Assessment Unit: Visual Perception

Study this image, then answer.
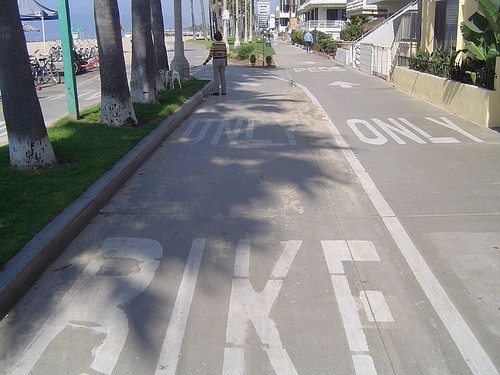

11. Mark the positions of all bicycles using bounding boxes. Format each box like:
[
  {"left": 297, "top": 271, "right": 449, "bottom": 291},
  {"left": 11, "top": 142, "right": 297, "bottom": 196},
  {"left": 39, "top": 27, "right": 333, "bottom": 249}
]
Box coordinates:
[{"left": 28, "top": 43, "right": 99, "bottom": 91}]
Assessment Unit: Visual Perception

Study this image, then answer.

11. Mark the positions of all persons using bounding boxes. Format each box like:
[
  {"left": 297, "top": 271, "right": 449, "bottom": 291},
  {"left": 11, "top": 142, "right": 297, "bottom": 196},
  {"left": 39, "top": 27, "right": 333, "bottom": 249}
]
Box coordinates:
[
  {"left": 202, "top": 31, "right": 228, "bottom": 96},
  {"left": 273, "top": 27, "right": 278, "bottom": 46},
  {"left": 304, "top": 29, "right": 314, "bottom": 53}
]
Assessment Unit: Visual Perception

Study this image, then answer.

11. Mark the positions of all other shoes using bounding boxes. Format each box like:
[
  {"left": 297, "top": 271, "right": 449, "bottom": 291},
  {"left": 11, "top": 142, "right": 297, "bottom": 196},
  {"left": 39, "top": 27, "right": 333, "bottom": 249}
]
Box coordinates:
[
  {"left": 221, "top": 92, "right": 227, "bottom": 95},
  {"left": 210, "top": 92, "right": 220, "bottom": 97}
]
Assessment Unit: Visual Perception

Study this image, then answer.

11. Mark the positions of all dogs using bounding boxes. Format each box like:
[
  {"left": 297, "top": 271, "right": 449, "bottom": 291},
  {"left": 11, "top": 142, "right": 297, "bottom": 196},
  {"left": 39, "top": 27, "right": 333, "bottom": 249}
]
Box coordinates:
[{"left": 164, "top": 69, "right": 181, "bottom": 90}]
